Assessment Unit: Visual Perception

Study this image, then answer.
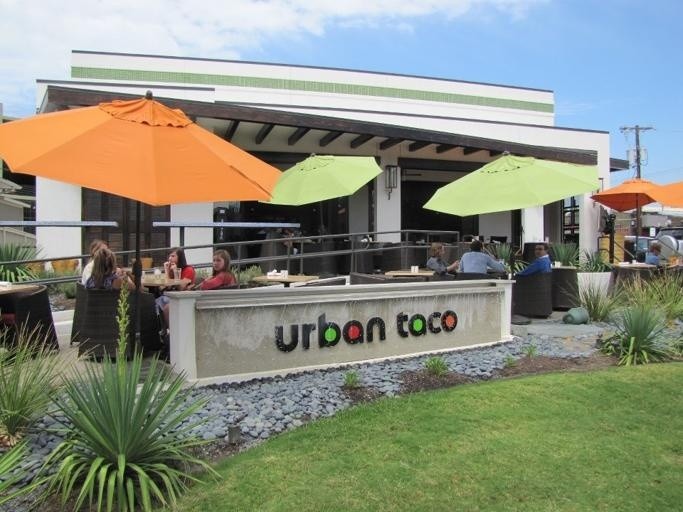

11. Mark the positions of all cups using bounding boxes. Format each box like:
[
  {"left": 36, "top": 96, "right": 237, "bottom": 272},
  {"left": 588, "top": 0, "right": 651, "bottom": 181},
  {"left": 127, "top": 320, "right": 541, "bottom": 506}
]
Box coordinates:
[
  {"left": 280, "top": 269, "right": 288, "bottom": 280},
  {"left": 114, "top": 268, "right": 126, "bottom": 277},
  {"left": 415, "top": 265, "right": 419, "bottom": 273},
  {"left": 142, "top": 270, "right": 161, "bottom": 282},
  {"left": 554, "top": 261, "right": 560, "bottom": 268},
  {"left": 173, "top": 267, "right": 181, "bottom": 281},
  {"left": 410, "top": 266, "right": 414, "bottom": 273},
  {"left": 632, "top": 260, "right": 636, "bottom": 264}
]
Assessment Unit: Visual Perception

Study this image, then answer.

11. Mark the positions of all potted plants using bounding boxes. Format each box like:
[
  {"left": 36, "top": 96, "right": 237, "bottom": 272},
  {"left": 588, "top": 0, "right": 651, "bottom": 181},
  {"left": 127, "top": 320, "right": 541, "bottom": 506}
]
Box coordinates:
[{"left": 578, "top": 249, "right": 611, "bottom": 310}]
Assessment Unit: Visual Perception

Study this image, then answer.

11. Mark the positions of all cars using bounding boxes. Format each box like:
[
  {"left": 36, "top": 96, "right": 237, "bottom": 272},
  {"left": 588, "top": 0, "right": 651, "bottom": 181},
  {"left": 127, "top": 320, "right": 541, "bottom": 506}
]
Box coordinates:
[{"left": 622, "top": 236, "right": 654, "bottom": 263}]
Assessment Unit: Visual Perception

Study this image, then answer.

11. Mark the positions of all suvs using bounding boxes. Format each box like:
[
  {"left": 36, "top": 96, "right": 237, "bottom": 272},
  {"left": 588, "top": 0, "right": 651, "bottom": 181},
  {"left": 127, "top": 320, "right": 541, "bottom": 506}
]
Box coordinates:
[{"left": 653, "top": 226, "right": 682, "bottom": 255}]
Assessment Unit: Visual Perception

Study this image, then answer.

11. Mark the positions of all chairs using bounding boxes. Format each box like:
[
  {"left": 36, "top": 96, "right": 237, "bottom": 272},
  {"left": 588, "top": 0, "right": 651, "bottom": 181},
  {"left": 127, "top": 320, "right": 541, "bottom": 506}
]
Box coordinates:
[
  {"left": 613, "top": 263, "right": 681, "bottom": 298},
  {"left": 352, "top": 271, "right": 425, "bottom": 290},
  {"left": 551, "top": 267, "right": 581, "bottom": 311},
  {"left": 0, "top": 272, "right": 249, "bottom": 361},
  {"left": 510, "top": 270, "right": 552, "bottom": 318},
  {"left": 289, "top": 277, "right": 348, "bottom": 291}
]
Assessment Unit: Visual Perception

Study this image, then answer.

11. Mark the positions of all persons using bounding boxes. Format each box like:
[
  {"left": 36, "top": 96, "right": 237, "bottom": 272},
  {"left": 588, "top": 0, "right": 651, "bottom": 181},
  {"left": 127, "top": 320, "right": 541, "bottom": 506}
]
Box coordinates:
[
  {"left": 515, "top": 243, "right": 552, "bottom": 274},
  {"left": 645, "top": 243, "right": 662, "bottom": 265},
  {"left": 426, "top": 241, "right": 460, "bottom": 274},
  {"left": 461, "top": 240, "right": 506, "bottom": 274},
  {"left": 80, "top": 240, "right": 237, "bottom": 327}
]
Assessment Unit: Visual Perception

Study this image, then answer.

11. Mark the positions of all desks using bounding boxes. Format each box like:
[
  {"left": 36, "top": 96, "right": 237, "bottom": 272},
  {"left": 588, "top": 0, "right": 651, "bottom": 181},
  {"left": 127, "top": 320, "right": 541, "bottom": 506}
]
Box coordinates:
[
  {"left": 256, "top": 272, "right": 317, "bottom": 284},
  {"left": 382, "top": 269, "right": 442, "bottom": 284}
]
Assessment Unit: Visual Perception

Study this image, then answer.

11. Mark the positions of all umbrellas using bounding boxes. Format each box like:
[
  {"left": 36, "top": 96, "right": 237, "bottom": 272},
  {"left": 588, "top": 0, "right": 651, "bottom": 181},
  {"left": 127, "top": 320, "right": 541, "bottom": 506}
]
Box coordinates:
[
  {"left": 258, "top": 153, "right": 384, "bottom": 235},
  {"left": 0, "top": 90, "right": 283, "bottom": 353},
  {"left": 422, "top": 152, "right": 600, "bottom": 218},
  {"left": 657, "top": 182, "right": 683, "bottom": 208},
  {"left": 591, "top": 179, "right": 660, "bottom": 249}
]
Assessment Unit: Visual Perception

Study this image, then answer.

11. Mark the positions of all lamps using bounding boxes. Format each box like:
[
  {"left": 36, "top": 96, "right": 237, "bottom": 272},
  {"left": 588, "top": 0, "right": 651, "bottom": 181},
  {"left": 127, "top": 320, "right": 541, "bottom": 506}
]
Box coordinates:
[{"left": 383, "top": 163, "right": 398, "bottom": 200}]
